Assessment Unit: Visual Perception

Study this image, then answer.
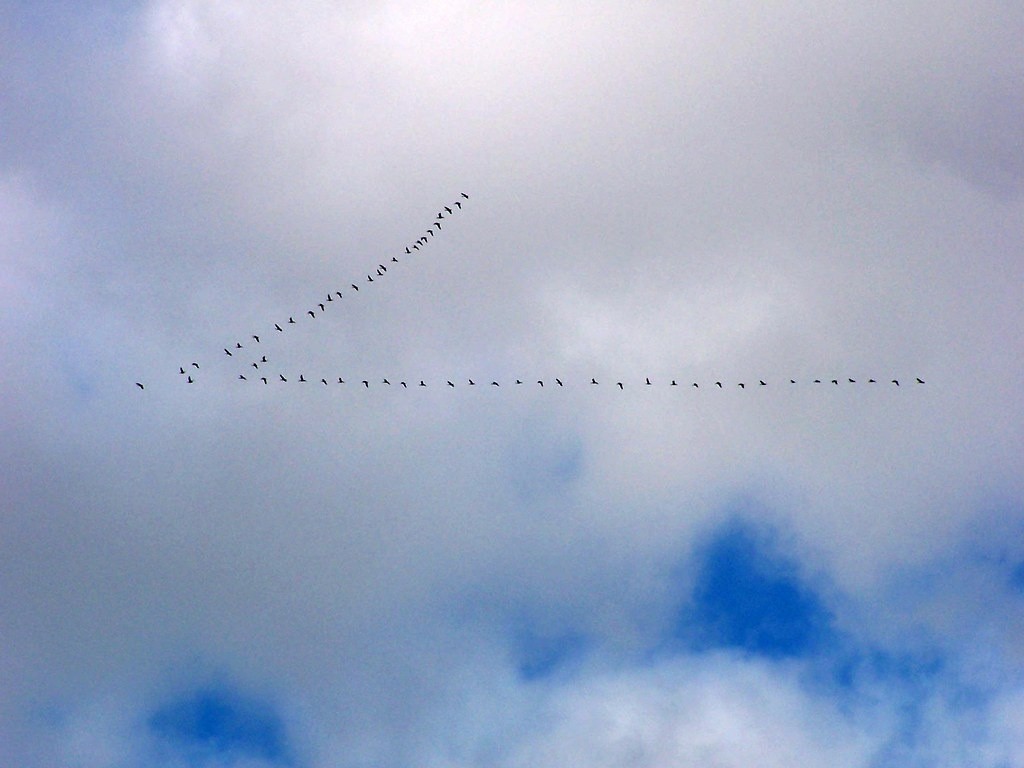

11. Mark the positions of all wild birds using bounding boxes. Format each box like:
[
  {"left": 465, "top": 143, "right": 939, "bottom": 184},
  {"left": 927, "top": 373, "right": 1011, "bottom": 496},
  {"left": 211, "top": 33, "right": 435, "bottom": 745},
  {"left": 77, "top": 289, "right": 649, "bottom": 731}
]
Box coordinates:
[
  {"left": 136, "top": 349, "right": 268, "bottom": 390},
  {"left": 278, "top": 374, "right": 926, "bottom": 390},
  {"left": 236, "top": 192, "right": 469, "bottom": 348}
]
